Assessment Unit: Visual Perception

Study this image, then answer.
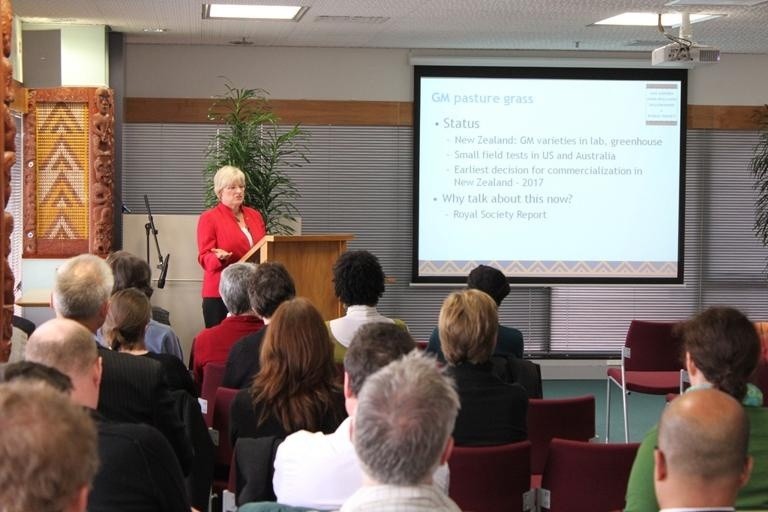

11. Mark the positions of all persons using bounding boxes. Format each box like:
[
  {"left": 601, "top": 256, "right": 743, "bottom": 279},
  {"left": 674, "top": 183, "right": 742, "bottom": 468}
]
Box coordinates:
[
  {"left": 0, "top": 246, "right": 531, "bottom": 512},
  {"left": 624, "top": 305, "right": 768, "bottom": 511},
  {"left": 196, "top": 164, "right": 268, "bottom": 329}
]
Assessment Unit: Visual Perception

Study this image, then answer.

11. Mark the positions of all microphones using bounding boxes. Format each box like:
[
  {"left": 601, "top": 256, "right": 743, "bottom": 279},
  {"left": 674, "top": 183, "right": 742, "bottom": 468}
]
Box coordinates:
[{"left": 158, "top": 253, "right": 169, "bottom": 288}]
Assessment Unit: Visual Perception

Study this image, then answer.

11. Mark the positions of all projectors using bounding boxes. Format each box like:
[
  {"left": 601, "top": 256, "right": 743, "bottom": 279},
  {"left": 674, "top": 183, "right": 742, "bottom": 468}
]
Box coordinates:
[{"left": 652, "top": 44, "right": 721, "bottom": 66}]
